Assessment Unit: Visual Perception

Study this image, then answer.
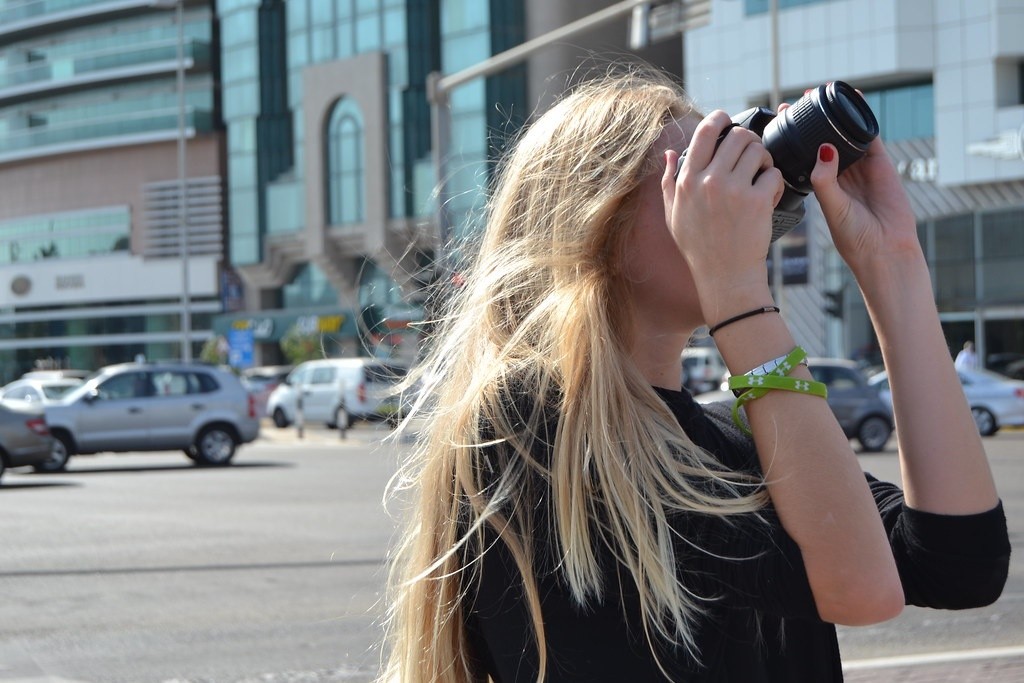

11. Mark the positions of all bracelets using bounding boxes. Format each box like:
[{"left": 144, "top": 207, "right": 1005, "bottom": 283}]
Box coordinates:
[
  {"left": 709, "top": 306, "right": 780, "bottom": 338},
  {"left": 729, "top": 345, "right": 829, "bottom": 440}
]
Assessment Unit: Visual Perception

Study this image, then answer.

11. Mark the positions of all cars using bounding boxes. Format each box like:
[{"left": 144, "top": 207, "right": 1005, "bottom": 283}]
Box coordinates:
[
  {"left": 867, "top": 361, "right": 1024, "bottom": 437},
  {"left": 715, "top": 355, "right": 895, "bottom": 452},
  {"left": 0, "top": 376, "right": 85, "bottom": 473}
]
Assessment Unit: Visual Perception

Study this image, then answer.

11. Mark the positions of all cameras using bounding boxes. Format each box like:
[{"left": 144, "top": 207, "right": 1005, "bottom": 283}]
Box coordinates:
[{"left": 711, "top": 79, "right": 880, "bottom": 244}]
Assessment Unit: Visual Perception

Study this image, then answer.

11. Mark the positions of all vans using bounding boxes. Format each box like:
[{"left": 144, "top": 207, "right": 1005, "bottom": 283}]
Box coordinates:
[{"left": 264, "top": 354, "right": 412, "bottom": 430}]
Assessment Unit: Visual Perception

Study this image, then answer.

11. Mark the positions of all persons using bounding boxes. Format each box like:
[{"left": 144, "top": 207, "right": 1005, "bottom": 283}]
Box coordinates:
[{"left": 375, "top": 79, "right": 1010, "bottom": 683}]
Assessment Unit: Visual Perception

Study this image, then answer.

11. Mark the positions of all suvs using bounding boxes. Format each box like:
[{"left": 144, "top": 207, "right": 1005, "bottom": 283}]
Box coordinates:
[{"left": 60, "top": 354, "right": 261, "bottom": 465}]
